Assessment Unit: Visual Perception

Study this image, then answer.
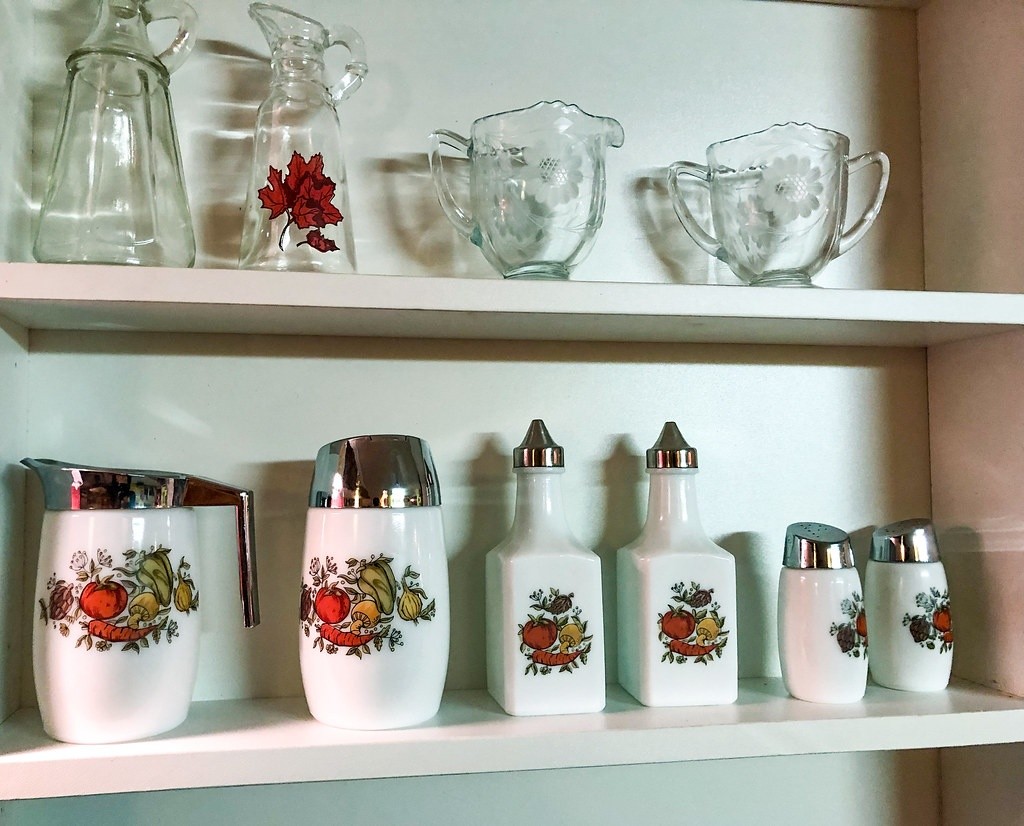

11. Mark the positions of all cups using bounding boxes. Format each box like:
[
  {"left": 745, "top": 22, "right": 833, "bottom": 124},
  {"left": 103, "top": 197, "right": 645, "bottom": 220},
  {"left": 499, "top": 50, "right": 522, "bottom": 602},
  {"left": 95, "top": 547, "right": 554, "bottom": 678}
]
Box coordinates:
[{"left": 667, "top": 120, "right": 891, "bottom": 288}]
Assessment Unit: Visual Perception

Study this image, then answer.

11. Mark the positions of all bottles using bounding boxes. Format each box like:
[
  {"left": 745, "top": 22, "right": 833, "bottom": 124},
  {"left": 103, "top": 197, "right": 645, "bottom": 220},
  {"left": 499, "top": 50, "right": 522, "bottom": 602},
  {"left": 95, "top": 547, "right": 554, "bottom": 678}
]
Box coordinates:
[
  {"left": 778, "top": 522, "right": 870, "bottom": 703},
  {"left": 484, "top": 420, "right": 605, "bottom": 717},
  {"left": 298, "top": 434, "right": 450, "bottom": 731},
  {"left": 864, "top": 518, "right": 954, "bottom": 693},
  {"left": 615, "top": 422, "right": 738, "bottom": 708}
]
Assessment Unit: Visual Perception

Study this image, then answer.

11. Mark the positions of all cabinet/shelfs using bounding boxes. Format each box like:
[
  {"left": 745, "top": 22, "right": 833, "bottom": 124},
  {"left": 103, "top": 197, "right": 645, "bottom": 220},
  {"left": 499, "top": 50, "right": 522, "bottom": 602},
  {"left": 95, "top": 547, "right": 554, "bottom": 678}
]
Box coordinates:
[{"left": 0, "top": 0, "right": 1024, "bottom": 826}]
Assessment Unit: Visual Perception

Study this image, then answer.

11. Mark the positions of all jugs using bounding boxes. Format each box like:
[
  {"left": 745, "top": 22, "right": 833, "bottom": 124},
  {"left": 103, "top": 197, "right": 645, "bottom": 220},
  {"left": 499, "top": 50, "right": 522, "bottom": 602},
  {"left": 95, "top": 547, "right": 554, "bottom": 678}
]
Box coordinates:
[
  {"left": 30, "top": 1, "right": 201, "bottom": 267},
  {"left": 428, "top": 100, "right": 626, "bottom": 282},
  {"left": 237, "top": 3, "right": 369, "bottom": 273},
  {"left": 18, "top": 457, "right": 260, "bottom": 745}
]
